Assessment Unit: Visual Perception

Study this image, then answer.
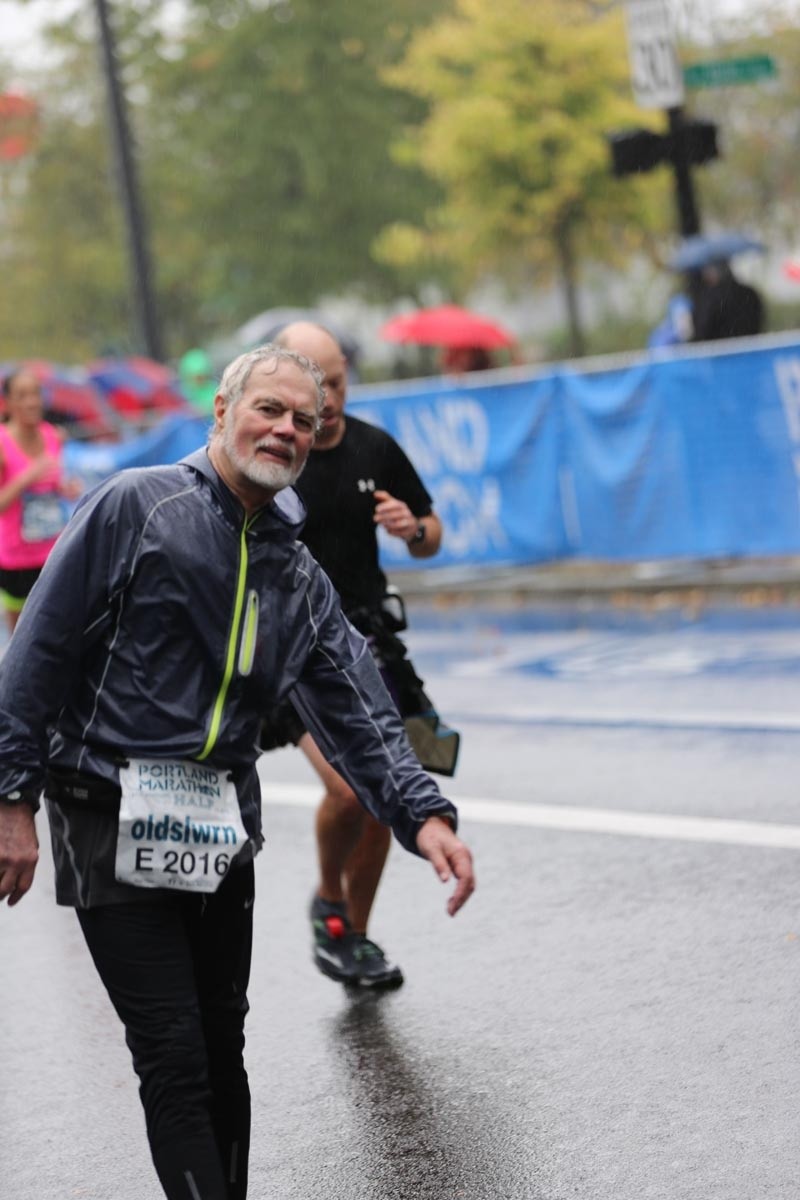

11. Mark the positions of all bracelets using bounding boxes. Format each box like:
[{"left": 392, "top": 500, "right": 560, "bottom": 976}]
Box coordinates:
[{"left": 407, "top": 521, "right": 426, "bottom": 544}]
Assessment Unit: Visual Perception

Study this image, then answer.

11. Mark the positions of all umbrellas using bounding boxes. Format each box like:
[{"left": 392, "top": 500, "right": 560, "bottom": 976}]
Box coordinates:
[
  {"left": 385, "top": 301, "right": 515, "bottom": 350},
  {"left": 663, "top": 226, "right": 768, "bottom": 265}
]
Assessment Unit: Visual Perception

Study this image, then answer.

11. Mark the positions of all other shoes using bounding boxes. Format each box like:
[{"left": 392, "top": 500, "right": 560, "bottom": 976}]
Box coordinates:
[
  {"left": 348, "top": 937, "right": 403, "bottom": 988},
  {"left": 309, "top": 894, "right": 363, "bottom": 981}
]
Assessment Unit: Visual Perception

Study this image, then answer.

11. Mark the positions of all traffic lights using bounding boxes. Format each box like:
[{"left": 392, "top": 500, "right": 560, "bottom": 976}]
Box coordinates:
[{"left": 609, "top": 120, "right": 724, "bottom": 182}]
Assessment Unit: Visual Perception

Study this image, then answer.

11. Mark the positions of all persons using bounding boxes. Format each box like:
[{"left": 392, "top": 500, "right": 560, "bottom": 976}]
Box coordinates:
[
  {"left": 668, "top": 259, "right": 767, "bottom": 341},
  {"left": 269, "top": 322, "right": 439, "bottom": 989},
  {"left": 0, "top": 344, "right": 479, "bottom": 1199},
  {"left": 434, "top": 348, "right": 496, "bottom": 375},
  {"left": 0, "top": 354, "right": 231, "bottom": 641}
]
